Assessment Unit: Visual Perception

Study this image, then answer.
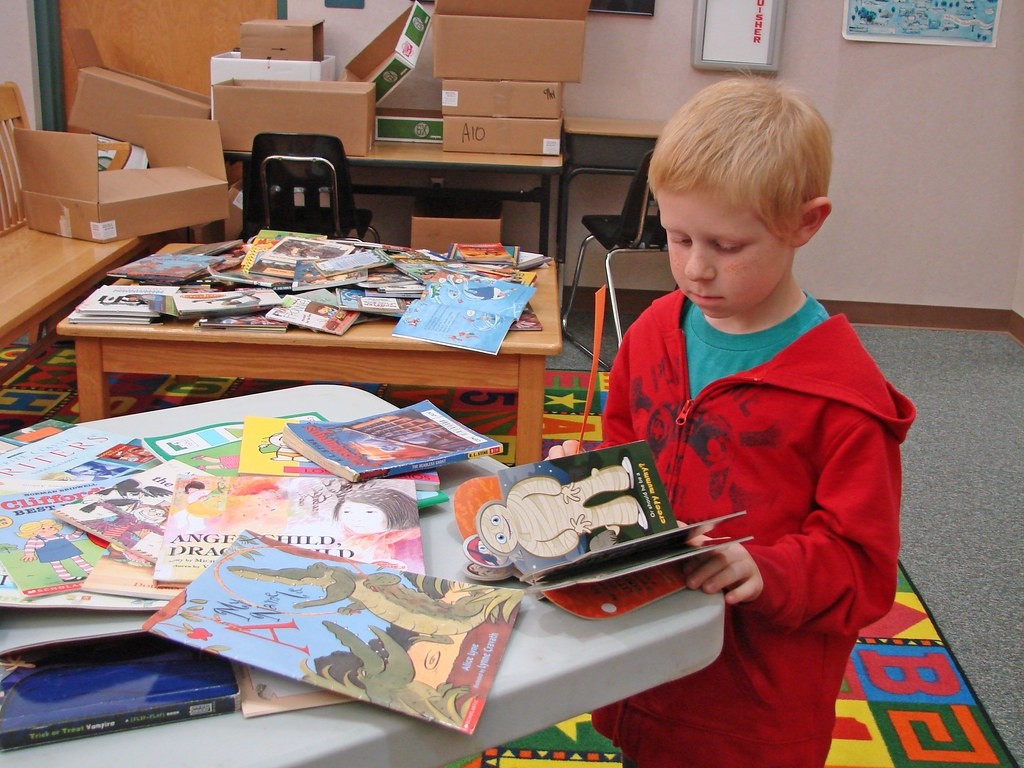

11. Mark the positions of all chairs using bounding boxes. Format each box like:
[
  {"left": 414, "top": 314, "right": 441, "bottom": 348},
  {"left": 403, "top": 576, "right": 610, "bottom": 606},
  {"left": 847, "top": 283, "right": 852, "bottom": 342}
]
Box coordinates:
[
  {"left": 557, "top": 150, "right": 679, "bottom": 367},
  {"left": 246, "top": 130, "right": 381, "bottom": 241}
]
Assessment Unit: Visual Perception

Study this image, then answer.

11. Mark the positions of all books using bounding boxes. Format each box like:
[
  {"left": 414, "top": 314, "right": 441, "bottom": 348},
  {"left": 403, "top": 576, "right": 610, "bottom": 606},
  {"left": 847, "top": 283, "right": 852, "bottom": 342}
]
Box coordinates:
[
  {"left": 0, "top": 398, "right": 755, "bottom": 752},
  {"left": 69, "top": 228, "right": 552, "bottom": 355}
]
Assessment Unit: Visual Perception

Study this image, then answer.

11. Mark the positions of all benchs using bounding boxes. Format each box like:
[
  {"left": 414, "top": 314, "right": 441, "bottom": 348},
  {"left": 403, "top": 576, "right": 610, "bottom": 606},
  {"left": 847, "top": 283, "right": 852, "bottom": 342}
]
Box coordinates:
[{"left": 2, "top": 83, "right": 138, "bottom": 354}]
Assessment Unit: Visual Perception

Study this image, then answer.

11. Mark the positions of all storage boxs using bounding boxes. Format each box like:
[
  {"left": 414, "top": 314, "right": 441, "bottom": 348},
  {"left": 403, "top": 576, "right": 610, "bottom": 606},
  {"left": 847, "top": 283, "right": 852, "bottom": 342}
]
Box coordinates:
[{"left": 15, "top": 1, "right": 591, "bottom": 242}]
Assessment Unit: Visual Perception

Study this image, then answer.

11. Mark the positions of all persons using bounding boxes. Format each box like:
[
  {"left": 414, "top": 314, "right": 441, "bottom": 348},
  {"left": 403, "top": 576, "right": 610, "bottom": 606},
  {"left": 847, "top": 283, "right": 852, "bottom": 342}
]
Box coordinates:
[{"left": 542, "top": 71, "right": 916, "bottom": 768}]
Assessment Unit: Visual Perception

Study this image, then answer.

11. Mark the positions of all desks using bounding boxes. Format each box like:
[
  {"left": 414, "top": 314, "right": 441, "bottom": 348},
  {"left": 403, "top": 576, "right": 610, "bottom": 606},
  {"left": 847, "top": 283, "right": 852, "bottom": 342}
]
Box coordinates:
[
  {"left": 222, "top": 143, "right": 565, "bottom": 258},
  {"left": 56, "top": 240, "right": 563, "bottom": 468},
  {"left": 0, "top": 385, "right": 725, "bottom": 768}
]
